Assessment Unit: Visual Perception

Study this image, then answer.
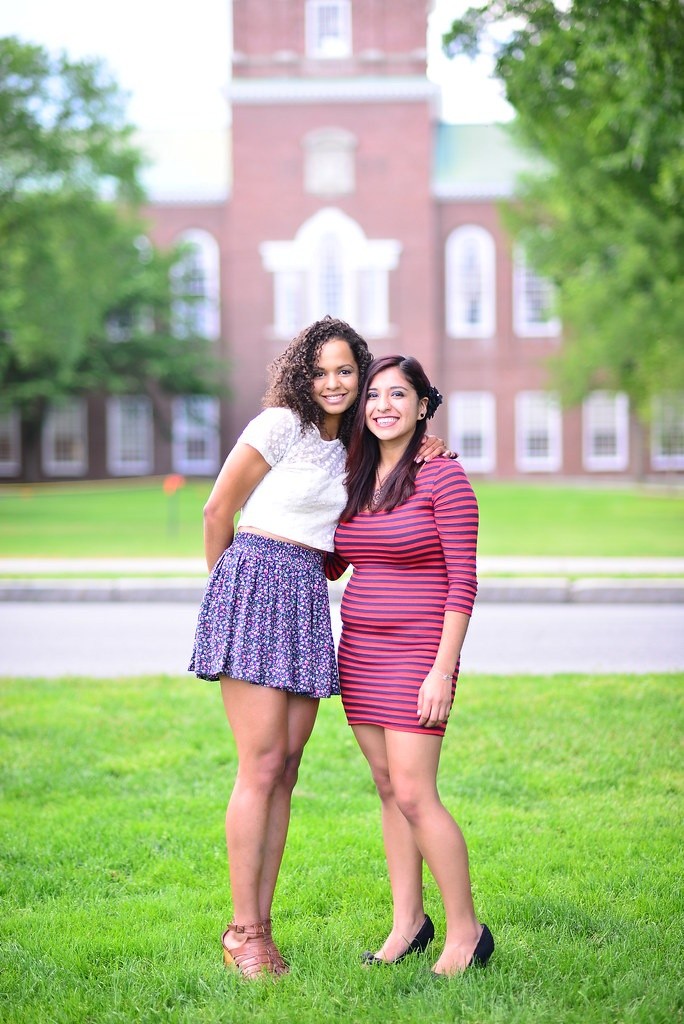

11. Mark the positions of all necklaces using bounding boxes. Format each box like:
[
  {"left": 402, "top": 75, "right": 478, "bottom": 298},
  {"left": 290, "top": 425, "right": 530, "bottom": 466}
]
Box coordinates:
[{"left": 373, "top": 466, "right": 390, "bottom": 506}]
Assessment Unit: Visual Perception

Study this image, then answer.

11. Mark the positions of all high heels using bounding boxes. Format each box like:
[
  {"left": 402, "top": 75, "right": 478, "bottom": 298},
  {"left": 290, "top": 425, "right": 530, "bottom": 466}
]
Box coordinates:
[
  {"left": 220, "top": 919, "right": 285, "bottom": 982},
  {"left": 428, "top": 924, "right": 495, "bottom": 983},
  {"left": 357, "top": 911, "right": 434, "bottom": 971}
]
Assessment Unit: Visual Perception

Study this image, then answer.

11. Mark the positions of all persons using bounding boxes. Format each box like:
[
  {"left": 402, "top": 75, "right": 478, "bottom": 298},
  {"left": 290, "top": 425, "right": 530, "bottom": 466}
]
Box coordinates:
[
  {"left": 187, "top": 317, "right": 451, "bottom": 980},
  {"left": 315, "top": 356, "right": 495, "bottom": 977}
]
